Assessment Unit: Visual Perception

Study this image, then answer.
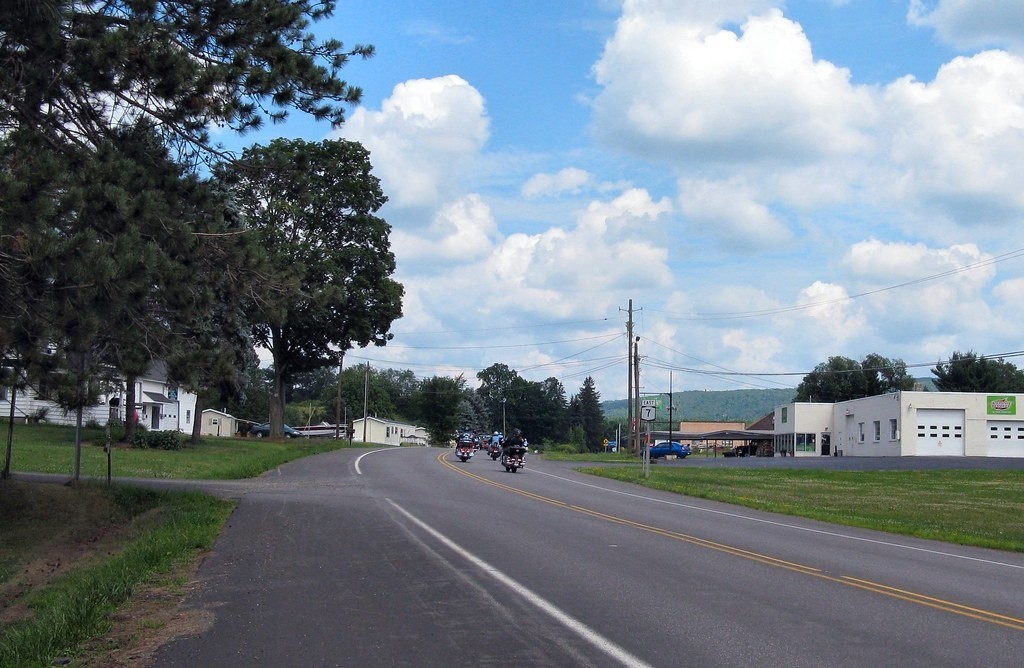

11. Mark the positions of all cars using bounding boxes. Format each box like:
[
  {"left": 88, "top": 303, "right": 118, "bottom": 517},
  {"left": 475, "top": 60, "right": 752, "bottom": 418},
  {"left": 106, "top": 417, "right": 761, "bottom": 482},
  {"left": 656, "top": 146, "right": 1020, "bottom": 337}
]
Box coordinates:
[
  {"left": 250, "top": 422, "right": 304, "bottom": 439},
  {"left": 737, "top": 445, "right": 757, "bottom": 457},
  {"left": 640, "top": 441, "right": 692, "bottom": 459}
]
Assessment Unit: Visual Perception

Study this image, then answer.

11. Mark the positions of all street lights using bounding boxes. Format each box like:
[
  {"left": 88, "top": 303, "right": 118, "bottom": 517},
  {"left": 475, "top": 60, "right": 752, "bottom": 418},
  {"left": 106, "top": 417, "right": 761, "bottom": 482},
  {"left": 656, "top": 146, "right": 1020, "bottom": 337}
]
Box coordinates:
[{"left": 502, "top": 398, "right": 506, "bottom": 438}]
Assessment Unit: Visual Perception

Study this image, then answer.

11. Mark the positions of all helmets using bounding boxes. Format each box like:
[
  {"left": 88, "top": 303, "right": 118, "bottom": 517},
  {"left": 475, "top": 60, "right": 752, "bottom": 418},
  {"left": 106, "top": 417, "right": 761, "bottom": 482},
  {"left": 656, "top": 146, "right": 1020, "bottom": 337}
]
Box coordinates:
[
  {"left": 494, "top": 431, "right": 499, "bottom": 435},
  {"left": 514, "top": 429, "right": 522, "bottom": 435}
]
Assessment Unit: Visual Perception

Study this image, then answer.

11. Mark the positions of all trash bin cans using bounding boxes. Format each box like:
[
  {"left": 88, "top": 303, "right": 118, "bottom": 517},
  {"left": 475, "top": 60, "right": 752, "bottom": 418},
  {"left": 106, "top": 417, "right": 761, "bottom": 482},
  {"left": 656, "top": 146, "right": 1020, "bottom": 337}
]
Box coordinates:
[
  {"left": 838, "top": 450, "right": 843, "bottom": 457},
  {"left": 780, "top": 450, "right": 787, "bottom": 457}
]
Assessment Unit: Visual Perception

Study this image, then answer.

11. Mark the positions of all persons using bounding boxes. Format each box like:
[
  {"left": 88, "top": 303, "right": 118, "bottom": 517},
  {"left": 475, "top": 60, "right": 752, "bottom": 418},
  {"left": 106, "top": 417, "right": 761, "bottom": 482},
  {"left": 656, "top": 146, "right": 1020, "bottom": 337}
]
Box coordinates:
[{"left": 451, "top": 426, "right": 529, "bottom": 463}]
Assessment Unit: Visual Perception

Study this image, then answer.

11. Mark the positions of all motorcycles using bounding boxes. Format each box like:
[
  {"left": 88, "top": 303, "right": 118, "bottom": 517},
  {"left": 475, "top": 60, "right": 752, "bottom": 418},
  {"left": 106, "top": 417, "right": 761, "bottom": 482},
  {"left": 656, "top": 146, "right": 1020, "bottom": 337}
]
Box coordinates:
[
  {"left": 487, "top": 440, "right": 504, "bottom": 461},
  {"left": 500, "top": 440, "right": 528, "bottom": 473},
  {"left": 454, "top": 430, "right": 478, "bottom": 462}
]
